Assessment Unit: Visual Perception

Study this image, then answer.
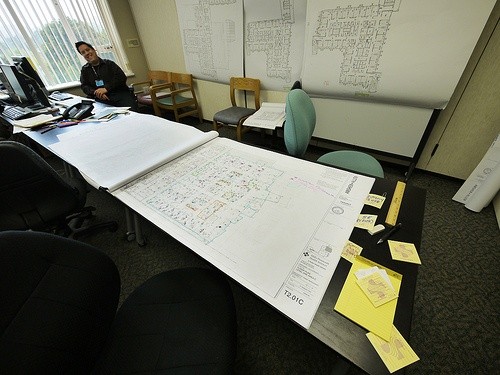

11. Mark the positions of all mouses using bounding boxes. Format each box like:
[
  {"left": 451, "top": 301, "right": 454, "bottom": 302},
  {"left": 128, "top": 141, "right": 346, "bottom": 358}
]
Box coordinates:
[{"left": 51, "top": 91, "right": 60, "bottom": 95}]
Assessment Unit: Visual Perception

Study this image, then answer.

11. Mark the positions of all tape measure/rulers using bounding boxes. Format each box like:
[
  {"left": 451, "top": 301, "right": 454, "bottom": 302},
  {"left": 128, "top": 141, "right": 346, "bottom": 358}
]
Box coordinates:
[{"left": 385, "top": 180, "right": 406, "bottom": 226}]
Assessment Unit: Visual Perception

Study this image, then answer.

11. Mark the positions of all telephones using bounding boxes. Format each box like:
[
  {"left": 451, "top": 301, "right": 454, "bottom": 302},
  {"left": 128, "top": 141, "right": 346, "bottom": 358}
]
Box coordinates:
[{"left": 62, "top": 99, "right": 96, "bottom": 120}]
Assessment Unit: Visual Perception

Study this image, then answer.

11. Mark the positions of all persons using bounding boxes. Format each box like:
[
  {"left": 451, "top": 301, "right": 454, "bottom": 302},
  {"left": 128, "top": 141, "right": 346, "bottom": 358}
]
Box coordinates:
[{"left": 75, "top": 40, "right": 137, "bottom": 113}]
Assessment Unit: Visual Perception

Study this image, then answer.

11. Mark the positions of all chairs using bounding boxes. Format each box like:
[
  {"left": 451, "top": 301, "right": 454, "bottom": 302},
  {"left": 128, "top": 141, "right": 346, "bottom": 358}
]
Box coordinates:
[
  {"left": 0, "top": 140, "right": 119, "bottom": 241},
  {"left": 250, "top": 81, "right": 303, "bottom": 153},
  {"left": 284, "top": 89, "right": 385, "bottom": 178},
  {"left": 0, "top": 231, "right": 239, "bottom": 375},
  {"left": 214, "top": 77, "right": 266, "bottom": 142},
  {"left": 131, "top": 70, "right": 201, "bottom": 125}
]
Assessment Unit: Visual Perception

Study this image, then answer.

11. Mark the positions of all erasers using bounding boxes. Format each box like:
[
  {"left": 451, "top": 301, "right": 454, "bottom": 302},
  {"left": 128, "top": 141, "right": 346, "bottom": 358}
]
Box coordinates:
[{"left": 368, "top": 225, "right": 385, "bottom": 236}]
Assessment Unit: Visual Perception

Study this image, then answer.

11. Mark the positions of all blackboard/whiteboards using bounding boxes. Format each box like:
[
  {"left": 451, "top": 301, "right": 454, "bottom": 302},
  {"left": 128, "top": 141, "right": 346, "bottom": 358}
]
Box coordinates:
[{"left": 245, "top": 88, "right": 441, "bottom": 166}]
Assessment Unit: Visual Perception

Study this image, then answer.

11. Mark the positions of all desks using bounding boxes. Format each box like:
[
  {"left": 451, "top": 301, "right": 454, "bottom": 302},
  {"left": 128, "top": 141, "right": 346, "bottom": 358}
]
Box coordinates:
[{"left": 0, "top": 91, "right": 428, "bottom": 375}]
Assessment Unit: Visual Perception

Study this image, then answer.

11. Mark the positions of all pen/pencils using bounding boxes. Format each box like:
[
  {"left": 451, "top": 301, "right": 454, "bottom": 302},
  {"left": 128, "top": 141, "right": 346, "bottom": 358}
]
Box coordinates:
[
  {"left": 375, "top": 221, "right": 403, "bottom": 245},
  {"left": 40, "top": 127, "right": 56, "bottom": 135}
]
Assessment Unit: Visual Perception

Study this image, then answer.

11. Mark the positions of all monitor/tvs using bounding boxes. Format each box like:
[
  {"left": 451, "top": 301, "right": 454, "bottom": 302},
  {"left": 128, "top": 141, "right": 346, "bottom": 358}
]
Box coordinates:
[
  {"left": 11, "top": 55, "right": 49, "bottom": 99},
  {"left": 0, "top": 64, "right": 34, "bottom": 100}
]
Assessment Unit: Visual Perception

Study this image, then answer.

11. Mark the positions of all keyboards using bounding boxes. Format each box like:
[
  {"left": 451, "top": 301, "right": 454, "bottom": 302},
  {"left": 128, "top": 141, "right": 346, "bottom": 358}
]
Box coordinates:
[
  {"left": 48, "top": 93, "right": 73, "bottom": 101},
  {"left": 2, "top": 105, "right": 31, "bottom": 120}
]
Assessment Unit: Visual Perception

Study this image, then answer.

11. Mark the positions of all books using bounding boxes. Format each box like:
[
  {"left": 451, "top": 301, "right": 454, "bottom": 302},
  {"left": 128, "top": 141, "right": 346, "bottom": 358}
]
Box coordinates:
[{"left": 9, "top": 103, "right": 131, "bottom": 130}]
